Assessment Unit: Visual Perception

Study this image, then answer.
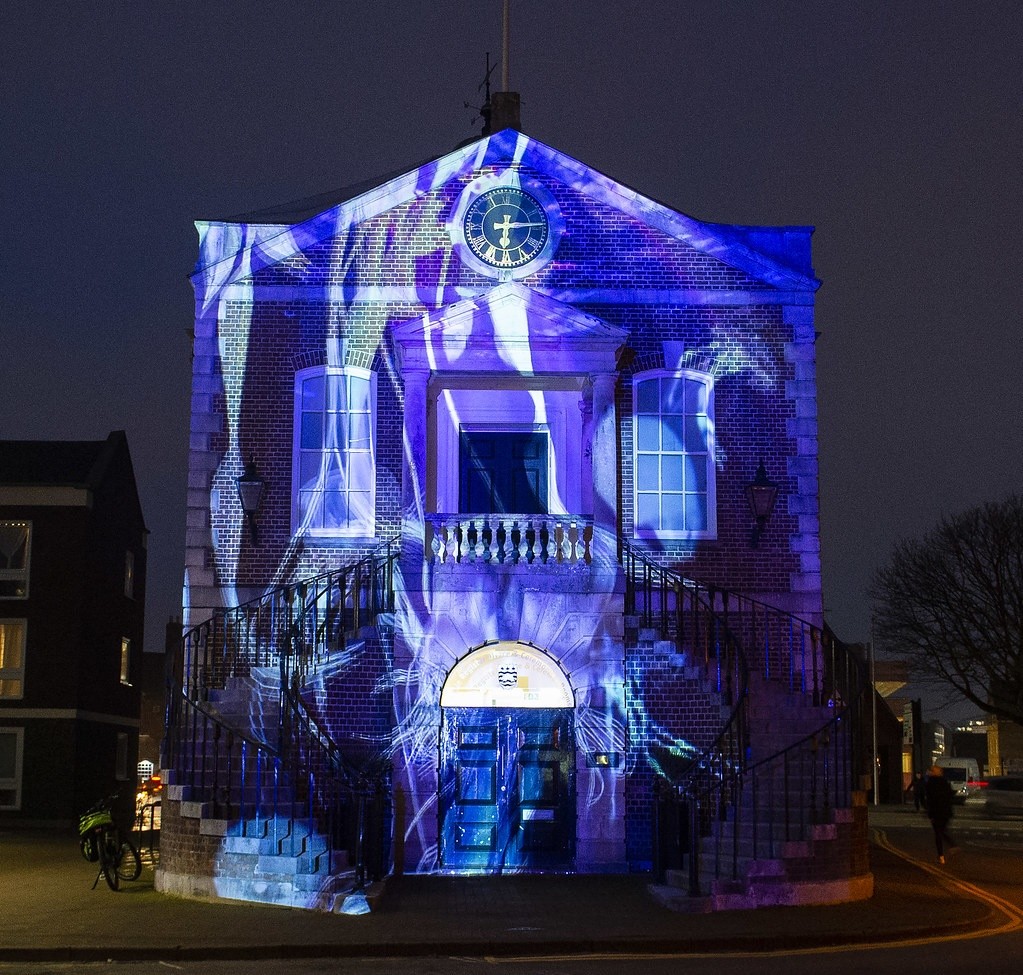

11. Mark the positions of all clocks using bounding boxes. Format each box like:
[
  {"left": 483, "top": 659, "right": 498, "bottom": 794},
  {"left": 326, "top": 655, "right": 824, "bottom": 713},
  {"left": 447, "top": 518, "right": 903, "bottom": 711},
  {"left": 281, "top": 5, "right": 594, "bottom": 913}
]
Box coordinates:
[{"left": 466, "top": 187, "right": 552, "bottom": 267}]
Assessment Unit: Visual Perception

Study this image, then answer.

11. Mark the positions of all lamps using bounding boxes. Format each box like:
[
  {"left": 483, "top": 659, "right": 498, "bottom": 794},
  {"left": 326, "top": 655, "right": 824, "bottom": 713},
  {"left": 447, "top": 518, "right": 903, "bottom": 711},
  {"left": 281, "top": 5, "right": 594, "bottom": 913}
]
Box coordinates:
[
  {"left": 233, "top": 464, "right": 267, "bottom": 546},
  {"left": 744, "top": 459, "right": 779, "bottom": 545}
]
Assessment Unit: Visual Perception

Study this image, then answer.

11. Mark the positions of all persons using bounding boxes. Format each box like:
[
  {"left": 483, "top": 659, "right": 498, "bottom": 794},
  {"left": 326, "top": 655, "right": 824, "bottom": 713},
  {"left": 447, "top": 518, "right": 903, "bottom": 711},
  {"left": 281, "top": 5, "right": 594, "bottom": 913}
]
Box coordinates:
[
  {"left": 917, "top": 764, "right": 967, "bottom": 866},
  {"left": 907, "top": 770, "right": 927, "bottom": 810}
]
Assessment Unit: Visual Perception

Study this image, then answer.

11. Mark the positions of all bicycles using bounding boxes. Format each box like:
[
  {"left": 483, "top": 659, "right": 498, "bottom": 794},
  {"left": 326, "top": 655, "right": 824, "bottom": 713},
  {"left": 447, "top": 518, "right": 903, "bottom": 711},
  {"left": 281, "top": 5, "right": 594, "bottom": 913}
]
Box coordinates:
[{"left": 78, "top": 788, "right": 142, "bottom": 891}]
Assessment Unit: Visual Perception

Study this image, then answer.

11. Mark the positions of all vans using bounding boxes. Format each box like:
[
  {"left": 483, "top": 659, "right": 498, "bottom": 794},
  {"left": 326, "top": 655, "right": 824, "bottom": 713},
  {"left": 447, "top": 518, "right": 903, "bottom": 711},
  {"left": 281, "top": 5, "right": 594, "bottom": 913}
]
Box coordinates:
[{"left": 933, "top": 758, "right": 982, "bottom": 805}]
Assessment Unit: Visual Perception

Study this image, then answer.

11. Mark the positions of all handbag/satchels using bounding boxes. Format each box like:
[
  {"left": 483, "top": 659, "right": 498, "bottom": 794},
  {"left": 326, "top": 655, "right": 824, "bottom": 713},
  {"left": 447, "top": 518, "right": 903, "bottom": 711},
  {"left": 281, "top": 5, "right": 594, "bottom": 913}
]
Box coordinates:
[
  {"left": 79, "top": 831, "right": 98, "bottom": 862},
  {"left": 78, "top": 808, "right": 111, "bottom": 836}
]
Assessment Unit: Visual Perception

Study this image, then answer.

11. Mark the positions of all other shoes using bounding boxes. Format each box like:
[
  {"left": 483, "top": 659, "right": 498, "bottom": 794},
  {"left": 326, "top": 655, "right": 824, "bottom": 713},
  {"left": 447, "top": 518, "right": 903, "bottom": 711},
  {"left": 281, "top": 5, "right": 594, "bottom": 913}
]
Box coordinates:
[{"left": 936, "top": 856, "right": 945, "bottom": 864}]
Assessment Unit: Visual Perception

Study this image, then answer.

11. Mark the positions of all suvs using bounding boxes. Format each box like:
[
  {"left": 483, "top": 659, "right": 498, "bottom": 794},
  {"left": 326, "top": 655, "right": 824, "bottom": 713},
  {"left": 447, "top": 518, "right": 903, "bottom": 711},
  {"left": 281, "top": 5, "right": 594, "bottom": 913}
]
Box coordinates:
[{"left": 965, "top": 775, "right": 1023, "bottom": 818}]
